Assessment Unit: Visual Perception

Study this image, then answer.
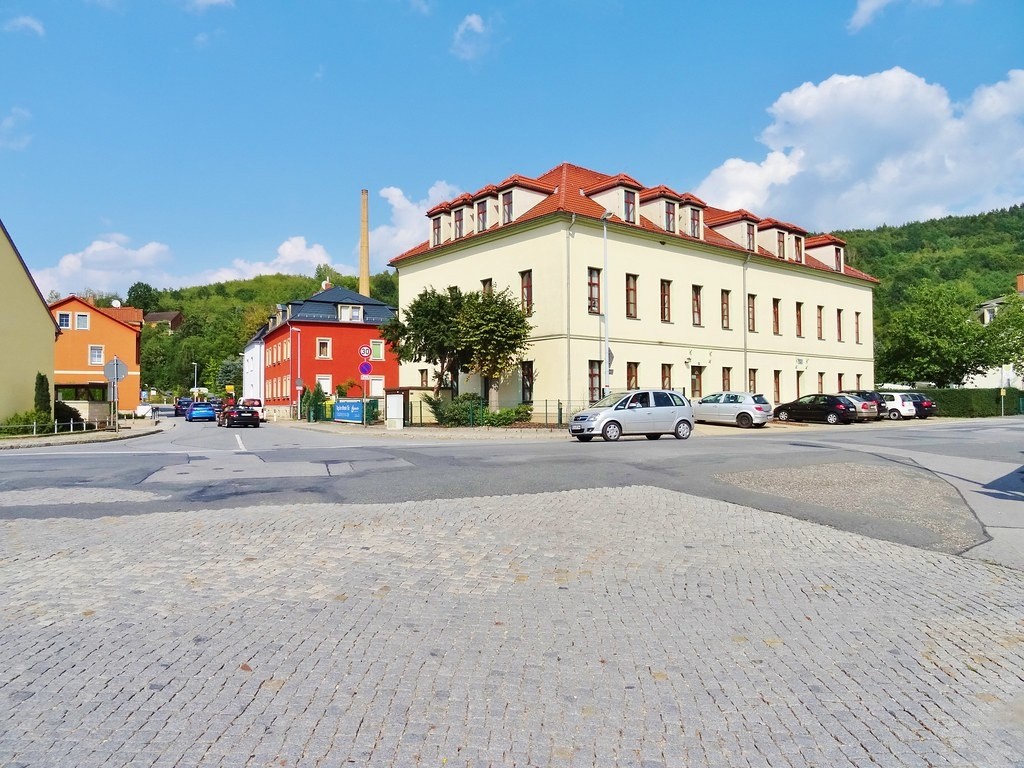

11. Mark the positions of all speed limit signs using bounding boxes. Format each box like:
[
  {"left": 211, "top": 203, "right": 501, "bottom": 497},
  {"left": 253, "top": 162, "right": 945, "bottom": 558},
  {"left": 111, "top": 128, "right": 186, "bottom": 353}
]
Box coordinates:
[{"left": 358, "top": 345, "right": 372, "bottom": 358}]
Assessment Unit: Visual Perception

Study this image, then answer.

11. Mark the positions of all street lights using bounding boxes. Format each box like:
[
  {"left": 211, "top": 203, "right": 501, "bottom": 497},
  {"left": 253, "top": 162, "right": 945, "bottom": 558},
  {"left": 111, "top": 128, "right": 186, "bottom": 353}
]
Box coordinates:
[
  {"left": 192, "top": 363, "right": 197, "bottom": 403},
  {"left": 292, "top": 327, "right": 300, "bottom": 420}
]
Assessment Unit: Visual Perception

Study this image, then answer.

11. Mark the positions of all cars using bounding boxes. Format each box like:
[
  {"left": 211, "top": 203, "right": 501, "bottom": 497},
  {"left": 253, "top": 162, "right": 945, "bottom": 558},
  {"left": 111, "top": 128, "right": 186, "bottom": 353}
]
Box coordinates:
[
  {"left": 832, "top": 394, "right": 878, "bottom": 424},
  {"left": 878, "top": 392, "right": 916, "bottom": 420},
  {"left": 173, "top": 399, "right": 192, "bottom": 417},
  {"left": 692, "top": 392, "right": 774, "bottom": 428},
  {"left": 908, "top": 393, "right": 938, "bottom": 420},
  {"left": 569, "top": 390, "right": 695, "bottom": 444},
  {"left": 774, "top": 394, "right": 858, "bottom": 425},
  {"left": 217, "top": 405, "right": 260, "bottom": 428},
  {"left": 185, "top": 402, "right": 216, "bottom": 422},
  {"left": 837, "top": 390, "right": 889, "bottom": 422},
  {"left": 210, "top": 399, "right": 224, "bottom": 413}
]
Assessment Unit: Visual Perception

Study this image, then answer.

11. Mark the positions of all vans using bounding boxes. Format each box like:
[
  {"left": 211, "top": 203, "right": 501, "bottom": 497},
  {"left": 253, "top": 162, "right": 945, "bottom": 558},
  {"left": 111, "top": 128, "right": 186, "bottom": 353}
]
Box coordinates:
[{"left": 238, "top": 398, "right": 264, "bottom": 422}]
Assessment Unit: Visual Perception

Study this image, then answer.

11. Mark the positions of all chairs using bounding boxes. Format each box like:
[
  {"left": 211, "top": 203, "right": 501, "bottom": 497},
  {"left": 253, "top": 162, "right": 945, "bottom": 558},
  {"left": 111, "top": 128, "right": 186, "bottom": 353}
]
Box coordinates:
[{"left": 640, "top": 397, "right": 647, "bottom": 407}]
